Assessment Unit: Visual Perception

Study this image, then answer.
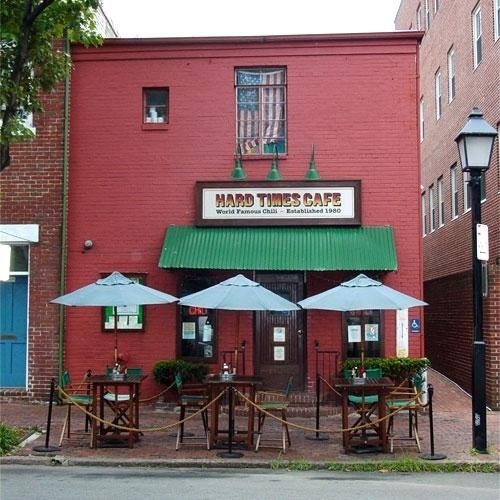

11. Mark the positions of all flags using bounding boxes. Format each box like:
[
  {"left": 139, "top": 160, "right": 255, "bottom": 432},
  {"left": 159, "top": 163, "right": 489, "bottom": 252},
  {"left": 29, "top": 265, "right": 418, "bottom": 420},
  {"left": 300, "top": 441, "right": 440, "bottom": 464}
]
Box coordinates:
[{"left": 235, "top": 69, "right": 284, "bottom": 155}]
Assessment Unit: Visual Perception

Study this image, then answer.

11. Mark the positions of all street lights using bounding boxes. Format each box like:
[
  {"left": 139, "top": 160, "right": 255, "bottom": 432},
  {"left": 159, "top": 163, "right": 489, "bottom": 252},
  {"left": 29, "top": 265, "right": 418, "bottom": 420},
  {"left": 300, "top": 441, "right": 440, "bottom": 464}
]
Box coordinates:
[{"left": 453, "top": 107, "right": 497, "bottom": 451}]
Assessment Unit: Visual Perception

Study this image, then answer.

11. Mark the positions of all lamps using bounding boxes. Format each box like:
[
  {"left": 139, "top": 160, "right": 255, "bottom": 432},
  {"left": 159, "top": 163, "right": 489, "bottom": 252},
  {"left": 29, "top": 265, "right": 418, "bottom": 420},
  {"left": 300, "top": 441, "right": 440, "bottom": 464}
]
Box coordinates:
[{"left": 229, "top": 140, "right": 323, "bottom": 182}]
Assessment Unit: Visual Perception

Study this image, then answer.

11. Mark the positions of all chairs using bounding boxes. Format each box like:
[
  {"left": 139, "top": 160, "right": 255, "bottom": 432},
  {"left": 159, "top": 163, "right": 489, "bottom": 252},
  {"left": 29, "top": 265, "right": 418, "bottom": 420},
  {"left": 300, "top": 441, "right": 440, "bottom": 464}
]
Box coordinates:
[
  {"left": 59, "top": 369, "right": 97, "bottom": 447},
  {"left": 103, "top": 367, "right": 144, "bottom": 439},
  {"left": 254, "top": 375, "right": 294, "bottom": 454},
  {"left": 384, "top": 383, "right": 425, "bottom": 453},
  {"left": 345, "top": 368, "right": 383, "bottom": 445},
  {"left": 174, "top": 372, "right": 211, "bottom": 451}
]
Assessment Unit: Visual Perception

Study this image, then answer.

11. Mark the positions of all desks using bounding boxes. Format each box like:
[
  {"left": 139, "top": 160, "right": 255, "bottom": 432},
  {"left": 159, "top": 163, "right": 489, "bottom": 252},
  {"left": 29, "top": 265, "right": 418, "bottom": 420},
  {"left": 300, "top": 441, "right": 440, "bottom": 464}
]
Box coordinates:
[
  {"left": 208, "top": 376, "right": 257, "bottom": 452},
  {"left": 89, "top": 375, "right": 139, "bottom": 450},
  {"left": 332, "top": 376, "right": 392, "bottom": 456}
]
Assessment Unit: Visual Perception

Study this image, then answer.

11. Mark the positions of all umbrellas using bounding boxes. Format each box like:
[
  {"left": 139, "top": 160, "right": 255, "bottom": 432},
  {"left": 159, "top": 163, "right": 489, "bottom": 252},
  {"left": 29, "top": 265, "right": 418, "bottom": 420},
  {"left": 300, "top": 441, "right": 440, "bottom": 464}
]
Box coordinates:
[
  {"left": 177, "top": 273, "right": 303, "bottom": 373},
  {"left": 295, "top": 273, "right": 432, "bottom": 374},
  {"left": 48, "top": 269, "right": 182, "bottom": 369}
]
